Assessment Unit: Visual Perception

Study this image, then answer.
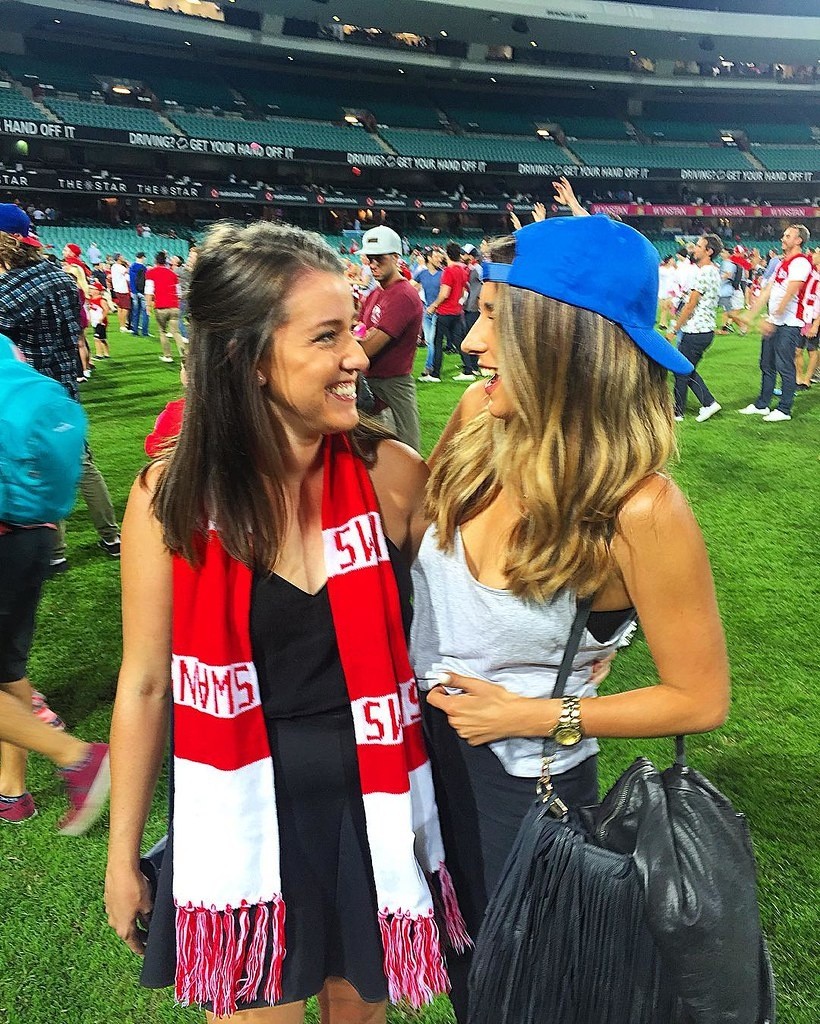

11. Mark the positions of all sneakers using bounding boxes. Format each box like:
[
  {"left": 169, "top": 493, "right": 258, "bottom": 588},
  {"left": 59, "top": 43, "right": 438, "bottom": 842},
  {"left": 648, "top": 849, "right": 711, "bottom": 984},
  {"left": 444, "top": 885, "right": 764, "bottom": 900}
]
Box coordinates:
[
  {"left": 762, "top": 408, "right": 790, "bottom": 422},
  {"left": 672, "top": 413, "right": 684, "bottom": 421},
  {"left": 739, "top": 404, "right": 769, "bottom": 415},
  {"left": 418, "top": 374, "right": 442, "bottom": 382},
  {"left": 452, "top": 370, "right": 484, "bottom": 383},
  {"left": 699, "top": 402, "right": 722, "bottom": 423}
]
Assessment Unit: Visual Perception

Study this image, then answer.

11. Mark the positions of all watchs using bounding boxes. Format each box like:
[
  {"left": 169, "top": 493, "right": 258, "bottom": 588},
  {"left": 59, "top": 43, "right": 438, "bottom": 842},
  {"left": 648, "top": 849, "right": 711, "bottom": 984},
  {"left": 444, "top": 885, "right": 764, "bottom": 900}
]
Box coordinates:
[{"left": 550, "top": 696, "right": 585, "bottom": 747}]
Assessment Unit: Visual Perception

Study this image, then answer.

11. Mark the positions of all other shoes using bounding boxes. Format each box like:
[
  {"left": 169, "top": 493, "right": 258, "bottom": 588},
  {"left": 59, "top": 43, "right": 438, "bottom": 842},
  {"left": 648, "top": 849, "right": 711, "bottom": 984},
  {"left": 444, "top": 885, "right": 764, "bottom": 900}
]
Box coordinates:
[
  {"left": 166, "top": 331, "right": 173, "bottom": 337},
  {"left": 47, "top": 554, "right": 67, "bottom": 569},
  {"left": 795, "top": 382, "right": 812, "bottom": 392},
  {"left": 64, "top": 744, "right": 111, "bottom": 840},
  {"left": 99, "top": 534, "right": 124, "bottom": 558},
  {"left": 83, "top": 369, "right": 91, "bottom": 377},
  {"left": 77, "top": 377, "right": 87, "bottom": 383},
  {"left": 657, "top": 322, "right": 668, "bottom": 333},
  {"left": 1, "top": 792, "right": 40, "bottom": 827},
  {"left": 182, "top": 336, "right": 191, "bottom": 344},
  {"left": 91, "top": 353, "right": 109, "bottom": 362},
  {"left": 159, "top": 355, "right": 174, "bottom": 364},
  {"left": 120, "top": 326, "right": 133, "bottom": 333}
]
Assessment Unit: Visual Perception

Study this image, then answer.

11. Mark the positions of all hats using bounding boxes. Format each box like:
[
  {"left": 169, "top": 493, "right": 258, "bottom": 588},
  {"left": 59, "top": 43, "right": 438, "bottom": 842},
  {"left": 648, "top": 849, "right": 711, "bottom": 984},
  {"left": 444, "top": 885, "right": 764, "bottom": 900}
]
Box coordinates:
[
  {"left": 67, "top": 243, "right": 82, "bottom": 255},
  {"left": 0, "top": 203, "right": 43, "bottom": 249},
  {"left": 353, "top": 225, "right": 402, "bottom": 256},
  {"left": 477, "top": 211, "right": 699, "bottom": 377},
  {"left": 460, "top": 245, "right": 478, "bottom": 256}
]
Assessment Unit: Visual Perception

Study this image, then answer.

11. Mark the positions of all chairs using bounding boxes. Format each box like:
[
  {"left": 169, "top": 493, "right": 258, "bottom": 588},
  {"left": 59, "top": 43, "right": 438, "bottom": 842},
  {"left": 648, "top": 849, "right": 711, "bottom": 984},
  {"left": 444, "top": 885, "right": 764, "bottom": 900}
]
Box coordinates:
[{"left": 1, "top": 92, "right": 820, "bottom": 271}]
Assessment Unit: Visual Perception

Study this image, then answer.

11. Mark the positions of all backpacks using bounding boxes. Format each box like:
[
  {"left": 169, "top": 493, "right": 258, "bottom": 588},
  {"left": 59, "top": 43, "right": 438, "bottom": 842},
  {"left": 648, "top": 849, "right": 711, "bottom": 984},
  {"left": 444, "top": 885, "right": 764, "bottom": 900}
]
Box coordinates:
[{"left": 727, "top": 261, "right": 743, "bottom": 288}]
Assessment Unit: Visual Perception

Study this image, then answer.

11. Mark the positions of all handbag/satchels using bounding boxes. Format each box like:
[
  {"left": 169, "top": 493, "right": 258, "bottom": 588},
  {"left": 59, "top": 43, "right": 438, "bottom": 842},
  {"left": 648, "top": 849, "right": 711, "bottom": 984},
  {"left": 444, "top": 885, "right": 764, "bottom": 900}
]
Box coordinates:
[{"left": 467, "top": 756, "right": 775, "bottom": 1023}]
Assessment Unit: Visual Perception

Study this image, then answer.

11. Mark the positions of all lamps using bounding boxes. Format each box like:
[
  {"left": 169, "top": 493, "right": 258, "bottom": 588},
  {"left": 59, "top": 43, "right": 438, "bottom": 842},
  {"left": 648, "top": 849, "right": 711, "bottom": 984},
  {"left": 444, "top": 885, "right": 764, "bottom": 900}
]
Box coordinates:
[
  {"left": 510, "top": 20, "right": 531, "bottom": 35},
  {"left": 698, "top": 35, "right": 715, "bottom": 51}
]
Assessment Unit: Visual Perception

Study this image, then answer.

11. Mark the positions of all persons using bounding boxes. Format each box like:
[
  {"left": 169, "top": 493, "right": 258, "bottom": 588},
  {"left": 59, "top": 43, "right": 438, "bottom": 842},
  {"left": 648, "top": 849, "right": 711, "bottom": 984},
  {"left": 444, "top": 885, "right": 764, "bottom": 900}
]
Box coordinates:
[
  {"left": 137, "top": 222, "right": 151, "bottom": 238},
  {"left": 353, "top": 225, "right": 423, "bottom": 451},
  {"left": 396, "top": 236, "right": 492, "bottom": 382},
  {"left": 656, "top": 223, "right": 748, "bottom": 423},
  {"left": 0, "top": 202, "right": 121, "bottom": 570},
  {"left": 341, "top": 242, "right": 358, "bottom": 255},
  {"left": 0, "top": 332, "right": 111, "bottom": 837},
  {"left": 738, "top": 225, "right": 820, "bottom": 421},
  {"left": 27, "top": 204, "right": 55, "bottom": 220},
  {"left": 552, "top": 176, "right": 590, "bottom": 216},
  {"left": 344, "top": 254, "right": 380, "bottom": 305},
  {"left": 510, "top": 201, "right": 546, "bottom": 229},
  {"left": 409, "top": 213, "right": 730, "bottom": 1024},
  {"left": 168, "top": 229, "right": 176, "bottom": 238},
  {"left": 58, "top": 241, "right": 200, "bottom": 383},
  {"left": 103, "top": 221, "right": 435, "bottom": 1024}
]
